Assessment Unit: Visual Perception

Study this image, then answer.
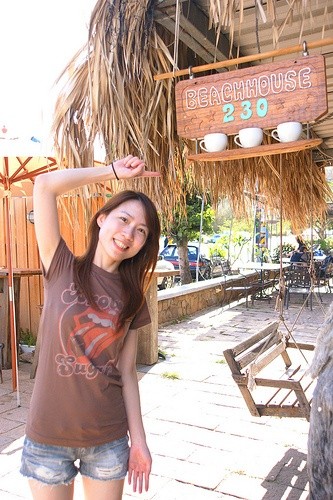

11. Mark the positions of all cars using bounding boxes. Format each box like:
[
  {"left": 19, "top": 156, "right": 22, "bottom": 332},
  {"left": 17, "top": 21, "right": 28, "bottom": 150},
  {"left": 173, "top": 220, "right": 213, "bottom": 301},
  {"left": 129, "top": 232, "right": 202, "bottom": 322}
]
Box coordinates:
[
  {"left": 150, "top": 254, "right": 176, "bottom": 290},
  {"left": 158, "top": 244, "right": 212, "bottom": 281}
]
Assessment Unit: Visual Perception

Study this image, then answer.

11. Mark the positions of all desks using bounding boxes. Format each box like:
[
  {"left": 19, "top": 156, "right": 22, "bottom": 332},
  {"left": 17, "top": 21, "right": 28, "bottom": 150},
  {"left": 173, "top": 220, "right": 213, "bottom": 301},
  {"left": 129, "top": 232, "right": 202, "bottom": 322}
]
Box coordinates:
[
  {"left": 134, "top": 269, "right": 181, "bottom": 365},
  {"left": 229, "top": 261, "right": 291, "bottom": 301},
  {"left": 278, "top": 256, "right": 328, "bottom": 286},
  {"left": 0, "top": 265, "right": 42, "bottom": 368}
]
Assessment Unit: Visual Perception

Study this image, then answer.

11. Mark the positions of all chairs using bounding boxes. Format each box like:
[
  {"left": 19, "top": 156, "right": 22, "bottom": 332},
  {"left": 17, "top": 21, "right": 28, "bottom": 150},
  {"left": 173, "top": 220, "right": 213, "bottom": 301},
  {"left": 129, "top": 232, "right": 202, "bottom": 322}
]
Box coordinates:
[{"left": 220, "top": 245, "right": 333, "bottom": 312}]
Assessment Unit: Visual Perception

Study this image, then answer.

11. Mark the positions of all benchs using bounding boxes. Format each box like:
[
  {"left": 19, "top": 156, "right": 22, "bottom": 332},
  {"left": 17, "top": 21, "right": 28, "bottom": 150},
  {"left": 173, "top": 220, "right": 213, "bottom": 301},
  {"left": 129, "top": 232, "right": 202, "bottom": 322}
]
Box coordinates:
[{"left": 222, "top": 320, "right": 316, "bottom": 423}]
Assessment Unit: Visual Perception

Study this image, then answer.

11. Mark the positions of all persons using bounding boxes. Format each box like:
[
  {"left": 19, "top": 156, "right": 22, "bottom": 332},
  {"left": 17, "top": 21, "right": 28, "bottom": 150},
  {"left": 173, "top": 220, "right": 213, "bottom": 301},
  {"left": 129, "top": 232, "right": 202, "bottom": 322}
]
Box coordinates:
[
  {"left": 17, "top": 152, "right": 162, "bottom": 500},
  {"left": 286, "top": 235, "right": 312, "bottom": 263}
]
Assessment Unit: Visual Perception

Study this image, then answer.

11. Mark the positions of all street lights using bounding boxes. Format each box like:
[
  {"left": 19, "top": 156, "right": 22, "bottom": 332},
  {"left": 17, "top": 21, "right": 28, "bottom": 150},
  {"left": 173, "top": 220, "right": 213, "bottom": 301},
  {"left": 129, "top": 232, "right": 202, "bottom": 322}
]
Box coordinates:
[{"left": 196, "top": 194, "right": 205, "bottom": 281}]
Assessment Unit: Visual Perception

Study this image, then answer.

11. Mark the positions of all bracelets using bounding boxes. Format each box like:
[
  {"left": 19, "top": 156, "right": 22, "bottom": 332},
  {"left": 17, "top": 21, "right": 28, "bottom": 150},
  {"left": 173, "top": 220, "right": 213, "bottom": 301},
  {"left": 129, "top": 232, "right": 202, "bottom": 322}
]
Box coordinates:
[{"left": 111, "top": 160, "right": 121, "bottom": 181}]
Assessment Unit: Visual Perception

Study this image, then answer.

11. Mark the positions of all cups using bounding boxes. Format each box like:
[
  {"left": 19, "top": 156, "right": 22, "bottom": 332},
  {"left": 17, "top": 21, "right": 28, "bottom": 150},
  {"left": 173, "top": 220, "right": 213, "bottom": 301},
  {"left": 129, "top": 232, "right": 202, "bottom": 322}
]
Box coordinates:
[
  {"left": 271, "top": 122, "right": 303, "bottom": 143},
  {"left": 234, "top": 127, "right": 264, "bottom": 148},
  {"left": 198, "top": 132, "right": 228, "bottom": 153}
]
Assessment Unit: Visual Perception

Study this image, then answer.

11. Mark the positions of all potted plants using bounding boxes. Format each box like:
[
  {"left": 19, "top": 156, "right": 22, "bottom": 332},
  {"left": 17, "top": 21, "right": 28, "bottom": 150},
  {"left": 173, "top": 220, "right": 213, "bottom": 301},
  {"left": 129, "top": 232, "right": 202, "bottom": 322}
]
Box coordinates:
[{"left": 19, "top": 327, "right": 37, "bottom": 353}]
